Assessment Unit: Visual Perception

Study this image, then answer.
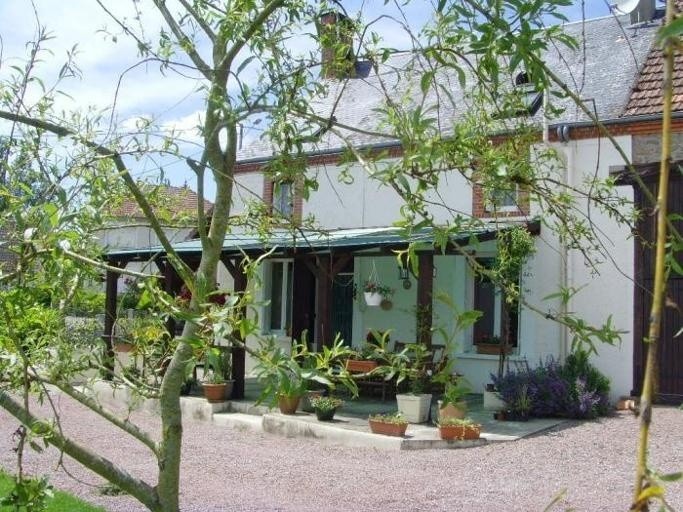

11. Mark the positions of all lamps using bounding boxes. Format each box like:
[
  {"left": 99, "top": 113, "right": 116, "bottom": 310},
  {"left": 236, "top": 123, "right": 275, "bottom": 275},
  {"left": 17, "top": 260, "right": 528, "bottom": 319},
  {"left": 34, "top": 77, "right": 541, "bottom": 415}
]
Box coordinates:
[{"left": 397, "top": 264, "right": 411, "bottom": 289}]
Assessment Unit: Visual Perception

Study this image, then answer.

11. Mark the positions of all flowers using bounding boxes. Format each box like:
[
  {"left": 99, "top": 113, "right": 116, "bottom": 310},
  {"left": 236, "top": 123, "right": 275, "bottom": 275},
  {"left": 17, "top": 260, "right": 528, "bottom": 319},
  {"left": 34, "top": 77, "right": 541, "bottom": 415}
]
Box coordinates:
[{"left": 352, "top": 282, "right": 394, "bottom": 300}]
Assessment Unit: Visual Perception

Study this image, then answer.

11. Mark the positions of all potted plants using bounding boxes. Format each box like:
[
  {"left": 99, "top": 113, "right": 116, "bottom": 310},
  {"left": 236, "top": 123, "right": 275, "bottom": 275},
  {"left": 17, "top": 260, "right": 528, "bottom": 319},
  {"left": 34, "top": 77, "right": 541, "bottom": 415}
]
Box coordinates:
[
  {"left": 202, "top": 371, "right": 234, "bottom": 402},
  {"left": 261, "top": 290, "right": 480, "bottom": 441},
  {"left": 469, "top": 225, "right": 535, "bottom": 408}
]
